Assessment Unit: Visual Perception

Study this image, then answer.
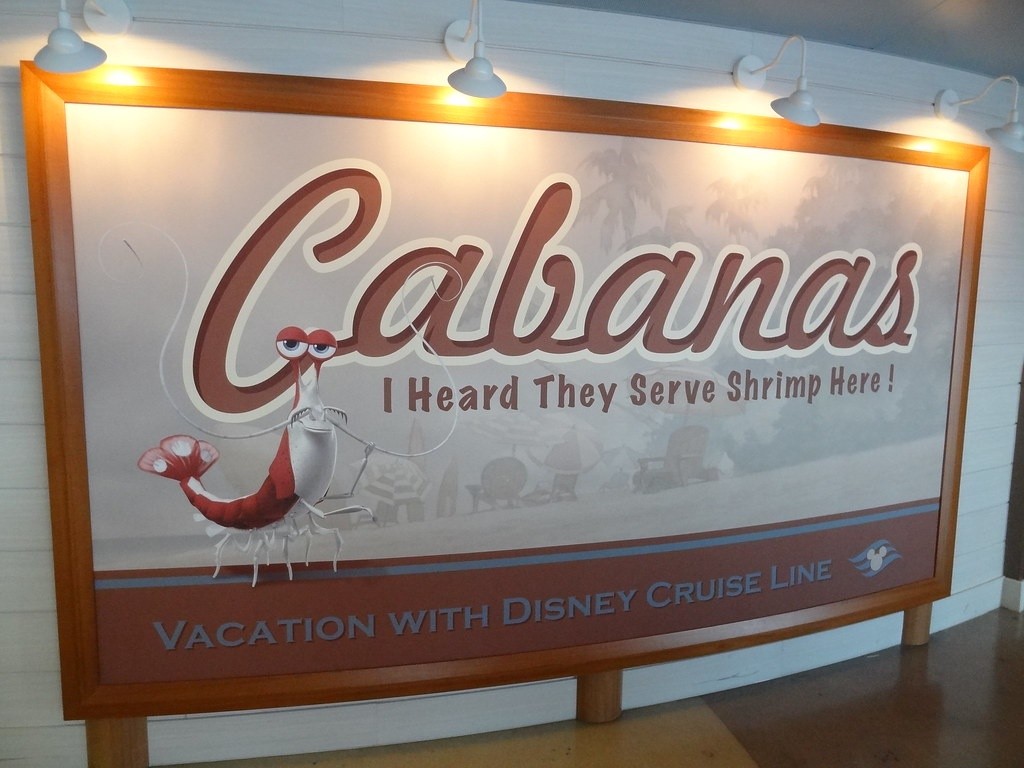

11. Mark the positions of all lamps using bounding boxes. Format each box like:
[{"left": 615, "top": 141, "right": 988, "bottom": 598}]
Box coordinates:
[
  {"left": 34, "top": 0, "right": 131, "bottom": 75},
  {"left": 441, "top": 0, "right": 508, "bottom": 99},
  {"left": 733, "top": 34, "right": 821, "bottom": 128},
  {"left": 934, "top": 74, "right": 1024, "bottom": 155}
]
